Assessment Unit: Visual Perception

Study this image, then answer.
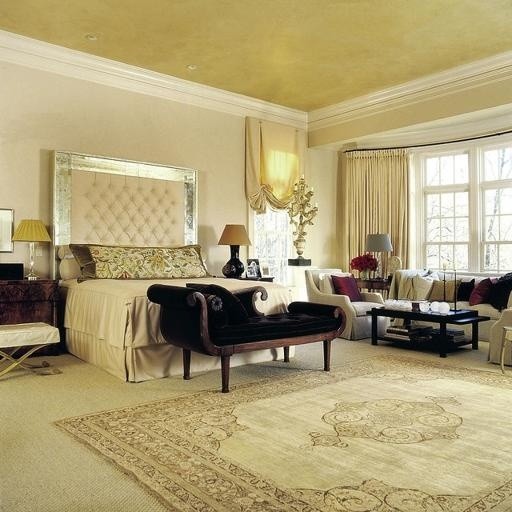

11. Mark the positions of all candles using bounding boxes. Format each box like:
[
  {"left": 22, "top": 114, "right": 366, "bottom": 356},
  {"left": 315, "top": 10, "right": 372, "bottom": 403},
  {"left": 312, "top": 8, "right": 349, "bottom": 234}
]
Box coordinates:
[{"left": 286, "top": 175, "right": 319, "bottom": 208}]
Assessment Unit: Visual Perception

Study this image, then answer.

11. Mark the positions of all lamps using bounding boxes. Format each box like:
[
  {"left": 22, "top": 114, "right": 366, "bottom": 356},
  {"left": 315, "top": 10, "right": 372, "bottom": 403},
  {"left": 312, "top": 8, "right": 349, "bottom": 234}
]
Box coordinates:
[
  {"left": 365, "top": 232, "right": 394, "bottom": 282},
  {"left": 12, "top": 219, "right": 53, "bottom": 279},
  {"left": 218, "top": 224, "right": 253, "bottom": 278}
]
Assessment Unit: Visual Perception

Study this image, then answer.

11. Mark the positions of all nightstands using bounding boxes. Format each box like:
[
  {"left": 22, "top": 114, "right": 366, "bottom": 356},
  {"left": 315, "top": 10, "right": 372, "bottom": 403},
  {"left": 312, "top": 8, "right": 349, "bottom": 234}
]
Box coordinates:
[
  {"left": 0, "top": 279, "right": 64, "bottom": 360},
  {"left": 240, "top": 276, "right": 275, "bottom": 282}
]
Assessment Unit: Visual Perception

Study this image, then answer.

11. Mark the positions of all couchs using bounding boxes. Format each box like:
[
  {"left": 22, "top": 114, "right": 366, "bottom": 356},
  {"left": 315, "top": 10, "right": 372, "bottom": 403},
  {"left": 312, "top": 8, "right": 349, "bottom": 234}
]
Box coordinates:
[{"left": 389, "top": 269, "right": 512, "bottom": 366}]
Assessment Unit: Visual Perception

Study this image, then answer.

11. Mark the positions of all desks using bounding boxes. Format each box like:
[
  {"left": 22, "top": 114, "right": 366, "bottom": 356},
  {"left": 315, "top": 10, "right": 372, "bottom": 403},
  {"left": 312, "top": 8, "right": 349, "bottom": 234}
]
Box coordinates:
[{"left": 356, "top": 277, "right": 390, "bottom": 300}]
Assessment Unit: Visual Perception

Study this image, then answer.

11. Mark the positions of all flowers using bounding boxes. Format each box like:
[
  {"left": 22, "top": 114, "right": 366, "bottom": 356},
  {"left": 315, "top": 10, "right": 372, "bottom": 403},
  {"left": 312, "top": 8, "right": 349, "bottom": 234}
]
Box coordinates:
[{"left": 351, "top": 254, "right": 378, "bottom": 272}]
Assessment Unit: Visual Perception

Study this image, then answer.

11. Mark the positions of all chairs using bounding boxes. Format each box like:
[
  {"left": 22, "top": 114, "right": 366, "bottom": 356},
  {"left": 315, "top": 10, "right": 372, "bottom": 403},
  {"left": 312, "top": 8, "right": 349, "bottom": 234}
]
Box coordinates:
[{"left": 305, "top": 269, "right": 389, "bottom": 340}]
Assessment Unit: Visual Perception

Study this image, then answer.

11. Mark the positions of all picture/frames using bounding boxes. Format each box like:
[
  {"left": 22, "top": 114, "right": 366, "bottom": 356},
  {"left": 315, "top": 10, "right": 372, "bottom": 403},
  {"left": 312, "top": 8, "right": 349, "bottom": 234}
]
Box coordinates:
[{"left": 0, "top": 208, "right": 14, "bottom": 253}]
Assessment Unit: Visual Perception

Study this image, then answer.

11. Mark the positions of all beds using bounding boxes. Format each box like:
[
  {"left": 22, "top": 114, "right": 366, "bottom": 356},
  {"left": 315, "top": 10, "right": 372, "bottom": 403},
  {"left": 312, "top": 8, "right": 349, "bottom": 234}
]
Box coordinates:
[{"left": 49, "top": 150, "right": 292, "bottom": 383}]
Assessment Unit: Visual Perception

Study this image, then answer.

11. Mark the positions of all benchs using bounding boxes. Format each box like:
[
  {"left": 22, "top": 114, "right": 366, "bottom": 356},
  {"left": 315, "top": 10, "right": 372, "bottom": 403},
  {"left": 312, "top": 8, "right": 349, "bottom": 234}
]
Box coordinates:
[{"left": 148, "top": 284, "right": 346, "bottom": 392}]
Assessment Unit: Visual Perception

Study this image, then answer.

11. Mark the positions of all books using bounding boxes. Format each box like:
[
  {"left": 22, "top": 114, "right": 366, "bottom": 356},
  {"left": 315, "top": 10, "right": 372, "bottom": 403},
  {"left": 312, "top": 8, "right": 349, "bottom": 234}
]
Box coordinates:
[{"left": 431, "top": 328, "right": 465, "bottom": 343}]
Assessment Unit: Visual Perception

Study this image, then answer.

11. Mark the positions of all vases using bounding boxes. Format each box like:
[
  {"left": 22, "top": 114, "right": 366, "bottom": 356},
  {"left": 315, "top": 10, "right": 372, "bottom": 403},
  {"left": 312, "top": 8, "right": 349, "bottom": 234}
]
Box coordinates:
[{"left": 360, "top": 269, "right": 370, "bottom": 281}]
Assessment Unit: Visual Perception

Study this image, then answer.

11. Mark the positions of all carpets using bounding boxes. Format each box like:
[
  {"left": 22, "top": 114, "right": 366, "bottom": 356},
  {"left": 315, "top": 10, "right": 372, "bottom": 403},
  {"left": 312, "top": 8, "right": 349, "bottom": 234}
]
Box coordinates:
[{"left": 55, "top": 355, "right": 512, "bottom": 512}]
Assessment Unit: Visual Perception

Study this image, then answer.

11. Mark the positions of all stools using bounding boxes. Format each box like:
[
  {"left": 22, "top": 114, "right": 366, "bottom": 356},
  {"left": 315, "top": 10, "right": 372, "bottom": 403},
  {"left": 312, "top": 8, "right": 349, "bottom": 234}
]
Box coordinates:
[{"left": 0, "top": 322, "right": 62, "bottom": 377}]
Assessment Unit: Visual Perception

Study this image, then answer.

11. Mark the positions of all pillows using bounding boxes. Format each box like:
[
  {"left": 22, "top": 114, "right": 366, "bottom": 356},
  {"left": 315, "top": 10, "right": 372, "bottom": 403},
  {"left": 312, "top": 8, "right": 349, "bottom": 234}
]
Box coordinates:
[
  {"left": 404, "top": 272, "right": 512, "bottom": 310},
  {"left": 184, "top": 283, "right": 251, "bottom": 326},
  {"left": 319, "top": 273, "right": 363, "bottom": 302},
  {"left": 58, "top": 243, "right": 213, "bottom": 284}
]
